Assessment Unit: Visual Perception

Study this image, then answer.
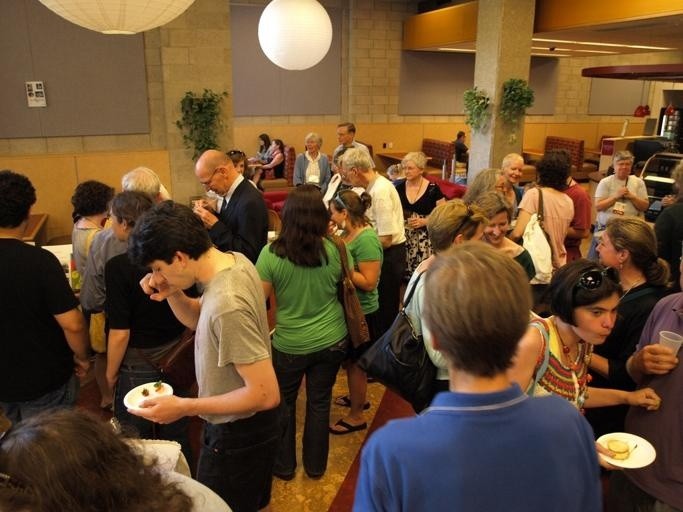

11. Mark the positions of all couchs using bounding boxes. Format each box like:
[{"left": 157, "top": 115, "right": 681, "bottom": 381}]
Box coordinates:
[
  {"left": 259, "top": 146, "right": 296, "bottom": 191},
  {"left": 423, "top": 137, "right": 456, "bottom": 179},
  {"left": 544, "top": 135, "right": 598, "bottom": 175},
  {"left": 424, "top": 176, "right": 468, "bottom": 200},
  {"left": 262, "top": 192, "right": 288, "bottom": 217},
  {"left": 355, "top": 139, "right": 373, "bottom": 160}
]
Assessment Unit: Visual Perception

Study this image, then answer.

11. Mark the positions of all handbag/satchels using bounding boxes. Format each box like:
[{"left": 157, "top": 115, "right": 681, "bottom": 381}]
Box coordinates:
[
  {"left": 325, "top": 232, "right": 371, "bottom": 353},
  {"left": 356, "top": 267, "right": 462, "bottom": 416},
  {"left": 521, "top": 182, "right": 552, "bottom": 285},
  {"left": 85, "top": 229, "right": 107, "bottom": 355}
]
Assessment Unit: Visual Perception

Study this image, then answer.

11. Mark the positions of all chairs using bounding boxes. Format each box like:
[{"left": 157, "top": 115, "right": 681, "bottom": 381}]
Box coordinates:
[{"left": 267, "top": 210, "right": 282, "bottom": 232}]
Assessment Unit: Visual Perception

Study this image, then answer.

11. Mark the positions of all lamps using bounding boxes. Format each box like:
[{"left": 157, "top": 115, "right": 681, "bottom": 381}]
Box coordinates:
[
  {"left": 37, "top": 0, "right": 196, "bottom": 35},
  {"left": 257, "top": 0, "right": 333, "bottom": 71}
]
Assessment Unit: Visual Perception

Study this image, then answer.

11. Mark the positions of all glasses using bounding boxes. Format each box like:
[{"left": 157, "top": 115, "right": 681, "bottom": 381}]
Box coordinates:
[
  {"left": 296, "top": 182, "right": 323, "bottom": 191},
  {"left": 199, "top": 167, "right": 219, "bottom": 192},
  {"left": 575, "top": 263, "right": 623, "bottom": 292}
]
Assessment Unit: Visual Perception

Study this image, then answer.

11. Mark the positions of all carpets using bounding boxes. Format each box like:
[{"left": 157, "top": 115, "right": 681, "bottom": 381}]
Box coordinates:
[{"left": 321, "top": 390, "right": 417, "bottom": 512}]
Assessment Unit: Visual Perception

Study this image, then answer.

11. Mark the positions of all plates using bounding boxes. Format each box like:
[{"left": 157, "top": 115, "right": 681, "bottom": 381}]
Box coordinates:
[
  {"left": 596, "top": 431, "right": 658, "bottom": 470},
  {"left": 124, "top": 380, "right": 174, "bottom": 409}
]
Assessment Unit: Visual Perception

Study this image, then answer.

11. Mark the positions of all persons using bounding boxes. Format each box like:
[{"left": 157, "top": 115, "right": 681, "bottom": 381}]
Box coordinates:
[
  {"left": 586, "top": 151, "right": 647, "bottom": 260},
  {"left": 339, "top": 150, "right": 403, "bottom": 332},
  {"left": 250, "top": 134, "right": 270, "bottom": 171},
  {"left": 389, "top": 201, "right": 486, "bottom": 416},
  {"left": 463, "top": 169, "right": 512, "bottom": 210},
  {"left": 254, "top": 185, "right": 354, "bottom": 480},
  {"left": 330, "top": 124, "right": 371, "bottom": 173},
  {"left": 495, "top": 155, "right": 526, "bottom": 210},
  {"left": 127, "top": 201, "right": 278, "bottom": 512},
  {"left": 191, "top": 150, "right": 271, "bottom": 328},
  {"left": 653, "top": 161, "right": 681, "bottom": 295},
  {"left": 359, "top": 242, "right": 601, "bottom": 512},
  {"left": 70, "top": 181, "right": 115, "bottom": 409},
  {"left": 584, "top": 217, "right": 669, "bottom": 512},
  {"left": 0, "top": 405, "right": 235, "bottom": 512},
  {"left": 392, "top": 153, "right": 441, "bottom": 289},
  {"left": 623, "top": 255, "right": 682, "bottom": 512},
  {"left": 260, "top": 140, "right": 285, "bottom": 178},
  {"left": 454, "top": 131, "right": 470, "bottom": 166},
  {"left": 105, "top": 192, "right": 201, "bottom": 476},
  {"left": 293, "top": 133, "right": 332, "bottom": 188},
  {"left": 510, "top": 154, "right": 575, "bottom": 316},
  {"left": 506, "top": 260, "right": 661, "bottom": 471},
  {"left": 470, "top": 190, "right": 539, "bottom": 314},
  {"left": 328, "top": 190, "right": 382, "bottom": 435},
  {"left": 553, "top": 151, "right": 591, "bottom": 261},
  {"left": 1, "top": 169, "right": 87, "bottom": 433},
  {"left": 121, "top": 167, "right": 167, "bottom": 205}
]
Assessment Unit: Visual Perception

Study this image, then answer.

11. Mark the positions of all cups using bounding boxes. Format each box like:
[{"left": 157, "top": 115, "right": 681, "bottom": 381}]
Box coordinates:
[{"left": 658, "top": 330, "right": 683, "bottom": 356}]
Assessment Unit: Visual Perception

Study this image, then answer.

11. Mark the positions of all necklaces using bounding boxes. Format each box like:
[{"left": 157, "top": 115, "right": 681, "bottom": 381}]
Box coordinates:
[{"left": 553, "top": 322, "right": 584, "bottom": 370}]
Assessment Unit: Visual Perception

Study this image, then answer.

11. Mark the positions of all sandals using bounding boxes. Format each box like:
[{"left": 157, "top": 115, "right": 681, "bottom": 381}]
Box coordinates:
[
  {"left": 327, "top": 416, "right": 369, "bottom": 435},
  {"left": 334, "top": 393, "right": 372, "bottom": 411}
]
